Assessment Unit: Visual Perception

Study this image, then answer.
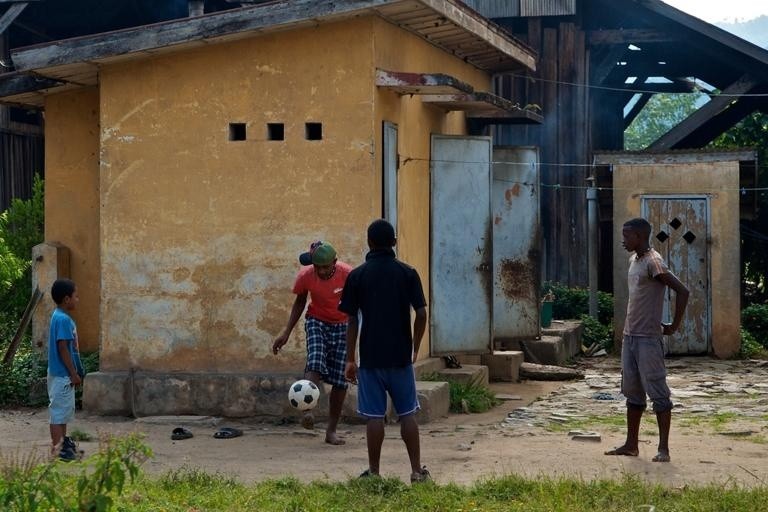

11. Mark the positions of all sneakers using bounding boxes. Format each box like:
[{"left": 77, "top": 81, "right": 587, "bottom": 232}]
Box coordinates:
[
  {"left": 52, "top": 437, "right": 81, "bottom": 460},
  {"left": 411, "top": 466, "right": 430, "bottom": 483},
  {"left": 361, "top": 470, "right": 380, "bottom": 478}
]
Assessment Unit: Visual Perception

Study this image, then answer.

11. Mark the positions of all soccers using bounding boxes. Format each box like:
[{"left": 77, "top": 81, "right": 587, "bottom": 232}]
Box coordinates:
[{"left": 288, "top": 380, "right": 319, "bottom": 411}]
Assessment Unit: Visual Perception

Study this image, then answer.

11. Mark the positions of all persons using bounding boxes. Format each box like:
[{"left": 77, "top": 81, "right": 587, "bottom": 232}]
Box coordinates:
[
  {"left": 272, "top": 241, "right": 354, "bottom": 446},
  {"left": 46, "top": 279, "right": 84, "bottom": 464},
  {"left": 604, "top": 218, "right": 689, "bottom": 463},
  {"left": 337, "top": 219, "right": 433, "bottom": 485}
]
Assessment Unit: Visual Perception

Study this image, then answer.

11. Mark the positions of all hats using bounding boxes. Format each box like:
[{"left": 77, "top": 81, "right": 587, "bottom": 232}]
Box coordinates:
[{"left": 300, "top": 241, "right": 336, "bottom": 266}]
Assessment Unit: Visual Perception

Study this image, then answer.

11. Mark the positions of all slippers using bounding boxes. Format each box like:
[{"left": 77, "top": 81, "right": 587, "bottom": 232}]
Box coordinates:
[
  {"left": 214, "top": 428, "right": 242, "bottom": 438},
  {"left": 172, "top": 428, "right": 193, "bottom": 440}
]
